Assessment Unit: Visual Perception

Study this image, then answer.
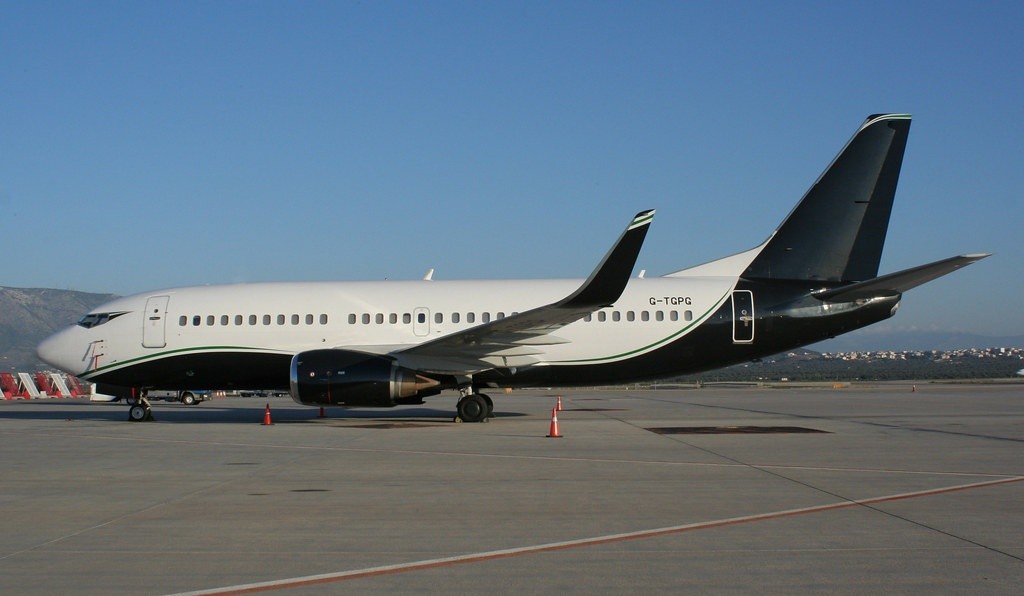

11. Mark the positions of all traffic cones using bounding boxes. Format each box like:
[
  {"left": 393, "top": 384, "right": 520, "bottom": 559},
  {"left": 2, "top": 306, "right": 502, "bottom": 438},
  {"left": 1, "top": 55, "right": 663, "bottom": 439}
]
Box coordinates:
[
  {"left": 556, "top": 396, "right": 562, "bottom": 411},
  {"left": 261, "top": 404, "right": 275, "bottom": 425},
  {"left": 546, "top": 407, "right": 563, "bottom": 438},
  {"left": 317, "top": 406, "right": 327, "bottom": 418}
]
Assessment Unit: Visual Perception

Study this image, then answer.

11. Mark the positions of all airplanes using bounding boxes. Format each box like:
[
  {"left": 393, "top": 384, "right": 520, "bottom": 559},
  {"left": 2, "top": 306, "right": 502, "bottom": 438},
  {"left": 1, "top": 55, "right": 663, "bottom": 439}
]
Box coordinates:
[{"left": 33, "top": 113, "right": 993, "bottom": 430}]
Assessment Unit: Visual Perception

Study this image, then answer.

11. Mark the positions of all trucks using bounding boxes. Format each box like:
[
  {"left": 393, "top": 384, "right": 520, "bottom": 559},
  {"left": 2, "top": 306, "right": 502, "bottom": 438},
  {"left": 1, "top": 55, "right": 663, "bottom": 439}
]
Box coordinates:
[{"left": 146, "top": 390, "right": 217, "bottom": 406}]
